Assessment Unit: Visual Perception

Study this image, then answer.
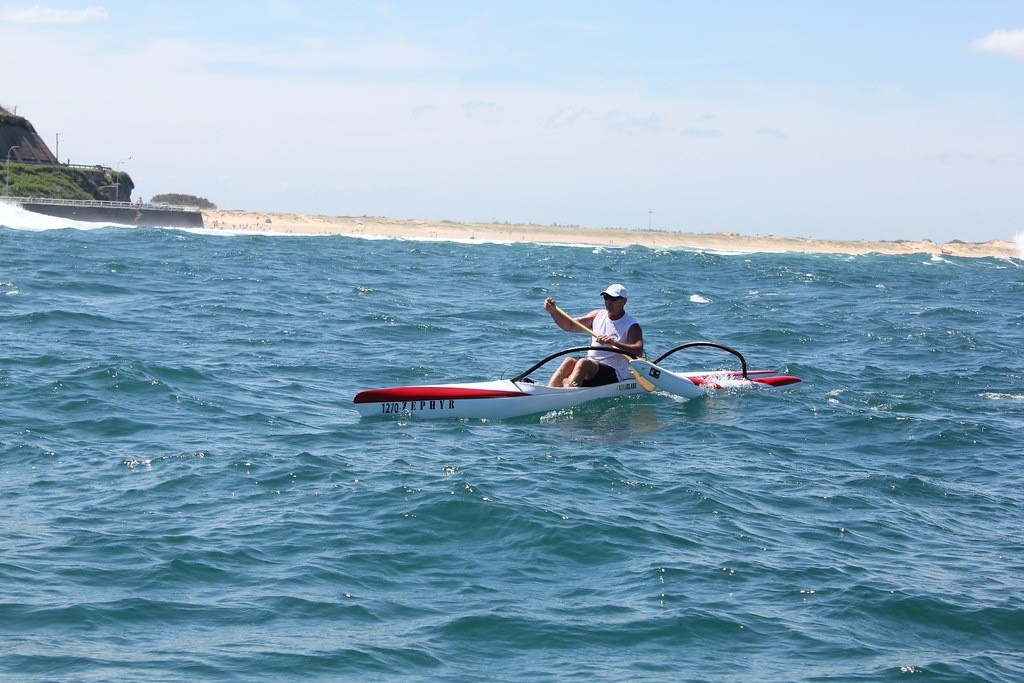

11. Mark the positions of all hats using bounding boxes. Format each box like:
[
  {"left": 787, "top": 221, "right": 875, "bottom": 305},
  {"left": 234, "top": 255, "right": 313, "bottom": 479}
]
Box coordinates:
[{"left": 600, "top": 284, "right": 627, "bottom": 298}]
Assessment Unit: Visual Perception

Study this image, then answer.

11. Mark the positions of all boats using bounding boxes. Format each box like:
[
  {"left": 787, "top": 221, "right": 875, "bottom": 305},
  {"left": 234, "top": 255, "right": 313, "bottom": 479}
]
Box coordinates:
[{"left": 352, "top": 343, "right": 803, "bottom": 418}]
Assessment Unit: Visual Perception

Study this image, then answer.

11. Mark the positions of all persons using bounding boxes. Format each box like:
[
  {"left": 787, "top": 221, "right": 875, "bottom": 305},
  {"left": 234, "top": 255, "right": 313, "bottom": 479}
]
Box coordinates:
[{"left": 544, "top": 283, "right": 644, "bottom": 388}]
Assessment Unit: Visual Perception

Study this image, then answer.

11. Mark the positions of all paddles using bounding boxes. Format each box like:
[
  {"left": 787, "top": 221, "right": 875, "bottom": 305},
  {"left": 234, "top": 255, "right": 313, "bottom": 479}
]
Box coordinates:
[{"left": 555, "top": 305, "right": 656, "bottom": 392}]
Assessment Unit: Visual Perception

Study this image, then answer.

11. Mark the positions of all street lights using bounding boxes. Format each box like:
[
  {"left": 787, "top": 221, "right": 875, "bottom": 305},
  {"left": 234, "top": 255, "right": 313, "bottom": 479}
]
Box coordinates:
[
  {"left": 56, "top": 133, "right": 63, "bottom": 161},
  {"left": 116, "top": 157, "right": 132, "bottom": 201},
  {"left": 6, "top": 146, "right": 20, "bottom": 197}
]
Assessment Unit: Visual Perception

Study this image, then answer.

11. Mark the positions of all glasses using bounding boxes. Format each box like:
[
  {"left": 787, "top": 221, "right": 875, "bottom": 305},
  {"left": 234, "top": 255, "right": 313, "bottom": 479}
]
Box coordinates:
[{"left": 604, "top": 295, "right": 619, "bottom": 301}]
